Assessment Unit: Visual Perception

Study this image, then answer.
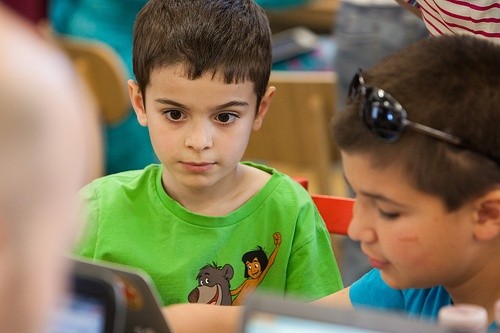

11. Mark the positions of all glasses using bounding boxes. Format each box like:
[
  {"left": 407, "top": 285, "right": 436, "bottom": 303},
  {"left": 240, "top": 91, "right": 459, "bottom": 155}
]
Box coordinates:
[{"left": 348, "top": 65, "right": 470, "bottom": 150}]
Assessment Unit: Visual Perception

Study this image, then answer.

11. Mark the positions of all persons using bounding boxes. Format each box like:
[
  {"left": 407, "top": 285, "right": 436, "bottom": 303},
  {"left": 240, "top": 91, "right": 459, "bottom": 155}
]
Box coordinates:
[
  {"left": 397, "top": 0, "right": 500, "bottom": 46},
  {"left": 0, "top": 0, "right": 105, "bottom": 333},
  {"left": 160, "top": 33, "right": 500, "bottom": 333},
  {"left": 71, "top": 0, "right": 345, "bottom": 306}
]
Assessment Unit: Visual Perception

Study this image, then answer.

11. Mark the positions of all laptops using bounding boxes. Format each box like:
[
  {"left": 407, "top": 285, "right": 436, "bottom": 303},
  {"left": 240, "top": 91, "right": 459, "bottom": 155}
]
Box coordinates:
[
  {"left": 68, "top": 256, "right": 173, "bottom": 333},
  {"left": 50, "top": 262, "right": 129, "bottom": 333},
  {"left": 234, "top": 295, "right": 450, "bottom": 332}
]
